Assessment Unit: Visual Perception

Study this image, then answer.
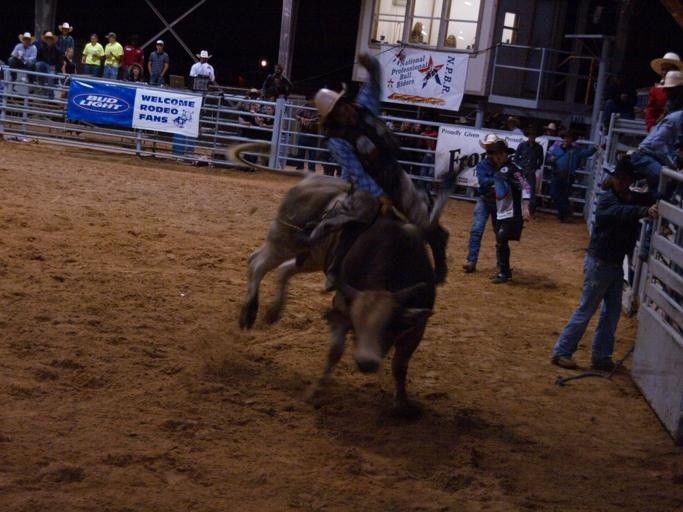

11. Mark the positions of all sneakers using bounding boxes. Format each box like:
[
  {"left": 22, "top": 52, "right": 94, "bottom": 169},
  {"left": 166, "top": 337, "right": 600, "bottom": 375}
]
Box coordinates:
[
  {"left": 552, "top": 353, "right": 578, "bottom": 371},
  {"left": 461, "top": 262, "right": 476, "bottom": 272},
  {"left": 590, "top": 357, "right": 627, "bottom": 373},
  {"left": 487, "top": 270, "right": 514, "bottom": 283}
]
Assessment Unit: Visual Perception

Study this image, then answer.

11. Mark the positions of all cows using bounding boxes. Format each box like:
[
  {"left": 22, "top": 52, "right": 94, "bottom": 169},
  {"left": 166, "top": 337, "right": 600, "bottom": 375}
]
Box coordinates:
[{"left": 226, "top": 141, "right": 437, "bottom": 413}]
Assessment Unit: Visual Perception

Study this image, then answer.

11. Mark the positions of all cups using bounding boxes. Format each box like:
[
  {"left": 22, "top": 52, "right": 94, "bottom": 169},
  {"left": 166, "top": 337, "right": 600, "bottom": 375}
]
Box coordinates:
[
  {"left": 92, "top": 48, "right": 96, "bottom": 58},
  {"left": 474, "top": 112, "right": 483, "bottom": 130},
  {"left": 18, "top": 51, "right": 22, "bottom": 59}
]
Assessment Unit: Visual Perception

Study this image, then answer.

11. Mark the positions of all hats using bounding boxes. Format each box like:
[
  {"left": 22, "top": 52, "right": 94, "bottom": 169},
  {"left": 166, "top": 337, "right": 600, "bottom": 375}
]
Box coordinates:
[
  {"left": 602, "top": 158, "right": 651, "bottom": 179},
  {"left": 486, "top": 141, "right": 516, "bottom": 156},
  {"left": 196, "top": 49, "right": 212, "bottom": 60},
  {"left": 313, "top": 82, "right": 350, "bottom": 132},
  {"left": 654, "top": 71, "right": 683, "bottom": 90},
  {"left": 246, "top": 87, "right": 262, "bottom": 98},
  {"left": 58, "top": 22, "right": 74, "bottom": 33},
  {"left": 104, "top": 31, "right": 117, "bottom": 40},
  {"left": 478, "top": 133, "right": 509, "bottom": 148},
  {"left": 19, "top": 32, "right": 36, "bottom": 44},
  {"left": 127, "top": 62, "right": 145, "bottom": 78},
  {"left": 41, "top": 31, "right": 59, "bottom": 43},
  {"left": 156, "top": 39, "right": 164, "bottom": 46},
  {"left": 543, "top": 122, "right": 558, "bottom": 132},
  {"left": 649, "top": 51, "right": 683, "bottom": 75}
]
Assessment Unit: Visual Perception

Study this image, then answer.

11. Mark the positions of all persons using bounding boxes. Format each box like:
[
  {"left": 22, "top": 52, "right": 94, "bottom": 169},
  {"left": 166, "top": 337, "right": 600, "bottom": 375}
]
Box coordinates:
[
  {"left": 410, "top": 23, "right": 456, "bottom": 48},
  {"left": 462, "top": 133, "right": 505, "bottom": 270},
  {"left": 8, "top": 23, "right": 215, "bottom": 115},
  {"left": 239, "top": 64, "right": 342, "bottom": 175},
  {"left": 376, "top": 108, "right": 601, "bottom": 221},
  {"left": 603, "top": 50, "right": 681, "bottom": 204},
  {"left": 313, "top": 51, "right": 448, "bottom": 292},
  {"left": 484, "top": 141, "right": 533, "bottom": 282},
  {"left": 549, "top": 156, "right": 664, "bottom": 374}
]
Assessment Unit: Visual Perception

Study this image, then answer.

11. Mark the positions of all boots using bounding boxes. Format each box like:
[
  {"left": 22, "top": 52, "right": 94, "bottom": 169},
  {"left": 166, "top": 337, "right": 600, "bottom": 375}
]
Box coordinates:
[
  {"left": 425, "top": 223, "right": 454, "bottom": 287},
  {"left": 322, "top": 218, "right": 359, "bottom": 293}
]
